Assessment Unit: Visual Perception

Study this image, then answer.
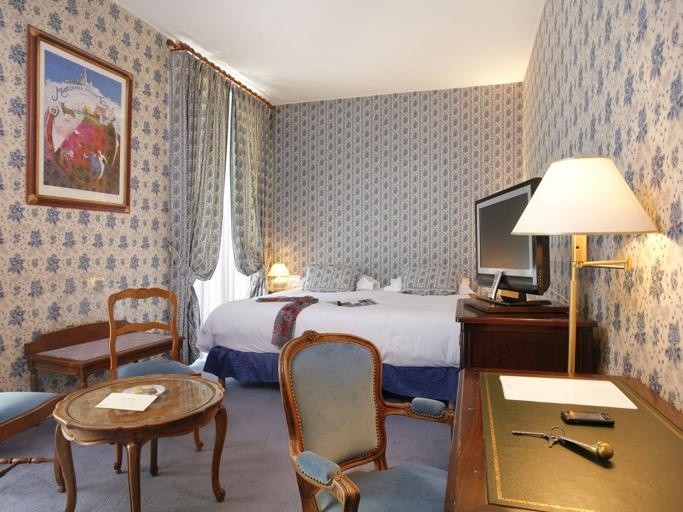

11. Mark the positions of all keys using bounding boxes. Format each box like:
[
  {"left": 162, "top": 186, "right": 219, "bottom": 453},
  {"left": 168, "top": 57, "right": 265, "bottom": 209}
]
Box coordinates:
[{"left": 512, "top": 426, "right": 614, "bottom": 460}]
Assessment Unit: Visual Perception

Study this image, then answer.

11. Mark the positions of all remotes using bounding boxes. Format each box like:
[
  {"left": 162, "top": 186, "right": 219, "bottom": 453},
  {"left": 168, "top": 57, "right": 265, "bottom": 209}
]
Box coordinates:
[{"left": 487, "top": 271, "right": 503, "bottom": 301}]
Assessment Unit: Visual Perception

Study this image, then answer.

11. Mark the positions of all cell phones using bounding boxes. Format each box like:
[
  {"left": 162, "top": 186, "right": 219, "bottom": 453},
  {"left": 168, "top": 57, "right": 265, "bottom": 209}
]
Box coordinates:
[{"left": 560, "top": 410, "right": 616, "bottom": 429}]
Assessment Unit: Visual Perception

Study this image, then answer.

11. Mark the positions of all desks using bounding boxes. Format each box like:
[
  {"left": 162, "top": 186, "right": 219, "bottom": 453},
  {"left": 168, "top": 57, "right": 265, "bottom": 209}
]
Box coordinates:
[
  {"left": 446, "top": 366, "right": 683, "bottom": 512},
  {"left": 51, "top": 372, "right": 229, "bottom": 512},
  {"left": 23, "top": 318, "right": 184, "bottom": 428}
]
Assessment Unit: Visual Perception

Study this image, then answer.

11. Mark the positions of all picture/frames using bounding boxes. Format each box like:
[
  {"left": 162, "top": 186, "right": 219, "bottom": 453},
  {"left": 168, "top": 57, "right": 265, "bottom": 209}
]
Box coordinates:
[{"left": 25, "top": 23, "right": 134, "bottom": 213}]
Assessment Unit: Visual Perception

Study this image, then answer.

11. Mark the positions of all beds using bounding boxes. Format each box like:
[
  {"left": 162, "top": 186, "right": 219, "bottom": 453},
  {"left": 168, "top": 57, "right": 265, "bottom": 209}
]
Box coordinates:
[{"left": 198, "top": 288, "right": 470, "bottom": 404}]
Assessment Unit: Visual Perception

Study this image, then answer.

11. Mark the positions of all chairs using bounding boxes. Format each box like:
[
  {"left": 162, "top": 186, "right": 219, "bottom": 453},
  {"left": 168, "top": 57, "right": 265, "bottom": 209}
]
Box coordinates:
[
  {"left": 276, "top": 330, "right": 454, "bottom": 512},
  {"left": 1, "top": 391, "right": 65, "bottom": 495},
  {"left": 108, "top": 288, "right": 203, "bottom": 480}
]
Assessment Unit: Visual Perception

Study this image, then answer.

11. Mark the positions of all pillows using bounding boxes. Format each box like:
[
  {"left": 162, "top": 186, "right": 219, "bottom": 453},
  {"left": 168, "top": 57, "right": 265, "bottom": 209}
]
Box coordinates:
[
  {"left": 301, "top": 262, "right": 366, "bottom": 292},
  {"left": 398, "top": 261, "right": 462, "bottom": 295}
]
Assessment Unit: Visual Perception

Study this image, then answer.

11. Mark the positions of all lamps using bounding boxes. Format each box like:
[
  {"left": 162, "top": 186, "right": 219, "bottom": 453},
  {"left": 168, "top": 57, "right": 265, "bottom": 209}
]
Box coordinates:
[
  {"left": 509, "top": 155, "right": 659, "bottom": 376},
  {"left": 267, "top": 263, "right": 289, "bottom": 293}
]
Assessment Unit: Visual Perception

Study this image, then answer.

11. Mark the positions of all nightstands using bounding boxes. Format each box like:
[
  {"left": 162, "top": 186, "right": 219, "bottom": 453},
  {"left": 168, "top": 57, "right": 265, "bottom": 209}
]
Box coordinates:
[{"left": 455, "top": 296, "right": 597, "bottom": 373}]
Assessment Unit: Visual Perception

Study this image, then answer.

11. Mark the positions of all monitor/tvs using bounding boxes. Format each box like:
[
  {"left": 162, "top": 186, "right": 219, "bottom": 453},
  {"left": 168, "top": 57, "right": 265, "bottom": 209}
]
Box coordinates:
[{"left": 474, "top": 177, "right": 552, "bottom": 306}]
